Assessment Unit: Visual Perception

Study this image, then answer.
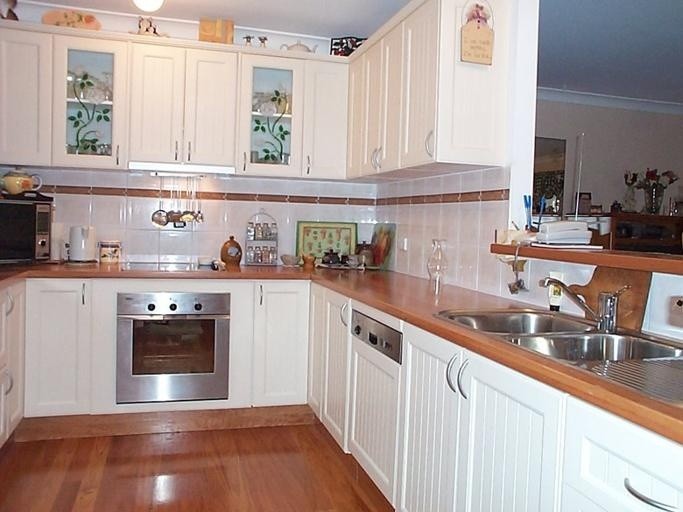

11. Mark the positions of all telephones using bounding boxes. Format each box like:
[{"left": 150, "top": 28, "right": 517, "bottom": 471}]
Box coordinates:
[{"left": 536, "top": 220, "right": 593, "bottom": 245}]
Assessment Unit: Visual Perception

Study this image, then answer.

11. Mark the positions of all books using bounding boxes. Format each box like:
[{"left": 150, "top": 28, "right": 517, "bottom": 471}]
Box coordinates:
[{"left": 531, "top": 241, "right": 603, "bottom": 250}]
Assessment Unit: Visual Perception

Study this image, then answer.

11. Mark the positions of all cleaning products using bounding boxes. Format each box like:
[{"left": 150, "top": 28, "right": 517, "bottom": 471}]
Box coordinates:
[{"left": 548, "top": 271, "right": 564, "bottom": 312}]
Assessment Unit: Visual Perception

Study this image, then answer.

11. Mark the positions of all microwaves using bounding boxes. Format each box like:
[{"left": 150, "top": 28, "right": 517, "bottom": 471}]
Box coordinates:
[{"left": 0, "top": 196, "right": 53, "bottom": 265}]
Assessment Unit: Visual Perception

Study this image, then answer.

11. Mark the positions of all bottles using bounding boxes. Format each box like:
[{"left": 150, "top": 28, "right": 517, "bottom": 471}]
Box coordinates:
[
  {"left": 247, "top": 245, "right": 277, "bottom": 265},
  {"left": 220, "top": 235, "right": 242, "bottom": 264},
  {"left": 68, "top": 223, "right": 97, "bottom": 261},
  {"left": 429, "top": 264, "right": 444, "bottom": 298},
  {"left": 99, "top": 240, "right": 121, "bottom": 264},
  {"left": 247, "top": 221, "right": 278, "bottom": 240},
  {"left": 225, "top": 265, "right": 241, "bottom": 273},
  {"left": 356, "top": 241, "right": 373, "bottom": 265},
  {"left": 427, "top": 237, "right": 450, "bottom": 282},
  {"left": 241, "top": 34, "right": 267, "bottom": 48}
]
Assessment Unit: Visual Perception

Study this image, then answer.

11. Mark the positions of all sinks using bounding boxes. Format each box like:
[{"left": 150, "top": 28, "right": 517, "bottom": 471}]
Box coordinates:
[
  {"left": 448, "top": 313, "right": 595, "bottom": 333},
  {"left": 499, "top": 333, "right": 683, "bottom": 362}
]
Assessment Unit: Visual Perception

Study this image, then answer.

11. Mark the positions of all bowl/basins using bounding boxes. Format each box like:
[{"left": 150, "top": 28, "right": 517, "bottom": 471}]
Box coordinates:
[{"left": 280, "top": 255, "right": 301, "bottom": 265}]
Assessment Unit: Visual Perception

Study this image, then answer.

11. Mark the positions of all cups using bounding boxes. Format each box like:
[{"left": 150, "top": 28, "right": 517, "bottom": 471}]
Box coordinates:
[
  {"left": 251, "top": 150, "right": 259, "bottom": 162},
  {"left": 302, "top": 251, "right": 315, "bottom": 268}
]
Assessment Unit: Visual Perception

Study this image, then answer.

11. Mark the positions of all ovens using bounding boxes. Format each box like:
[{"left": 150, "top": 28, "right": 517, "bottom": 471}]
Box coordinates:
[{"left": 116, "top": 292, "right": 229, "bottom": 403}]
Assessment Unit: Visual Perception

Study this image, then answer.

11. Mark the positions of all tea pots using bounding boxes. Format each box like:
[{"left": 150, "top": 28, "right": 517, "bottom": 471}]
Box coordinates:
[
  {"left": 1, "top": 166, "right": 42, "bottom": 194},
  {"left": 321, "top": 248, "right": 341, "bottom": 263},
  {"left": 280, "top": 39, "right": 318, "bottom": 54}
]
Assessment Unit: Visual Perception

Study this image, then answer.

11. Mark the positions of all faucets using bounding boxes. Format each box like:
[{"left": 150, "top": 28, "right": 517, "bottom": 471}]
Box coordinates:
[{"left": 543, "top": 277, "right": 632, "bottom": 334}]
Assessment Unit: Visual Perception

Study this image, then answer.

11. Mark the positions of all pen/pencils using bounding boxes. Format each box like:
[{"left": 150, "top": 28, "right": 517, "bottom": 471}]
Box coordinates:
[
  {"left": 512, "top": 221, "right": 519, "bottom": 231},
  {"left": 524, "top": 195, "right": 546, "bottom": 232}
]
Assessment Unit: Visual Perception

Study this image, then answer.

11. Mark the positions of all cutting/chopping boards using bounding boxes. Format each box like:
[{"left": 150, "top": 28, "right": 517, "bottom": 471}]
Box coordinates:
[{"left": 567, "top": 265, "right": 652, "bottom": 334}]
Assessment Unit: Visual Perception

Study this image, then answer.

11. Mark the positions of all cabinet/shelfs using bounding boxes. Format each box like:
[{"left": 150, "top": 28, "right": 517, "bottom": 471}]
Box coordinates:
[
  {"left": 0, "top": 279, "right": 311, "bottom": 444},
  {"left": 0, "top": 0, "right": 442, "bottom": 174},
  {"left": 307, "top": 281, "right": 683, "bottom": 511}
]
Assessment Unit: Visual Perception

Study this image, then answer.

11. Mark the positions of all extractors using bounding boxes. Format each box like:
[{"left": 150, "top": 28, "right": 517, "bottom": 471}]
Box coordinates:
[{"left": 128, "top": 161, "right": 236, "bottom": 179}]
